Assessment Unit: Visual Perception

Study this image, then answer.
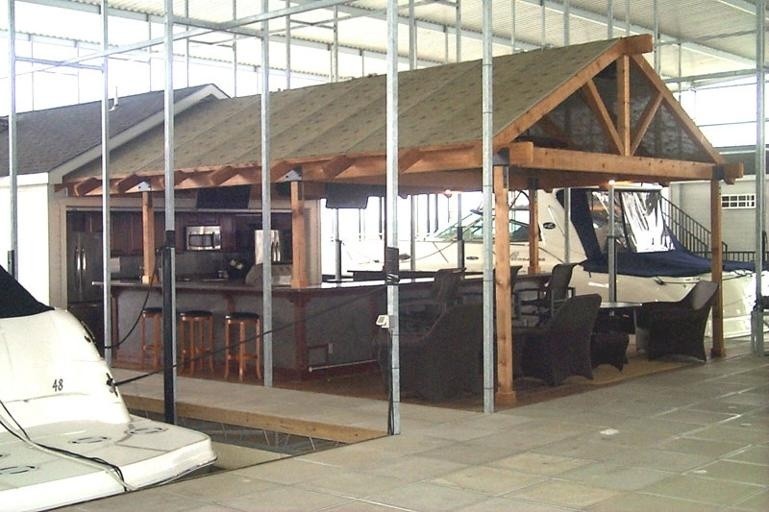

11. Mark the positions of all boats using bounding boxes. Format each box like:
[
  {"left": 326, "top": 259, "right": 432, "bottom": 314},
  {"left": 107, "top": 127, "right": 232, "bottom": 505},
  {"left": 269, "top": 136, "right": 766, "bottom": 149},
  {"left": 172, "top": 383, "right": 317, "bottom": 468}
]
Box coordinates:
[
  {"left": 0, "top": 264, "right": 220, "bottom": 512},
  {"left": 351, "top": 175, "right": 769, "bottom": 342}
]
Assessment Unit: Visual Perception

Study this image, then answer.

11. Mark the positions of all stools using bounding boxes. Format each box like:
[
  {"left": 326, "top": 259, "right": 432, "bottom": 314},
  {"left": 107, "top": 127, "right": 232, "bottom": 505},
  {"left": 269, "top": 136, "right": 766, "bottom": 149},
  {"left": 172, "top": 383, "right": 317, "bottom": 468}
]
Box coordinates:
[{"left": 138, "top": 306, "right": 262, "bottom": 383}]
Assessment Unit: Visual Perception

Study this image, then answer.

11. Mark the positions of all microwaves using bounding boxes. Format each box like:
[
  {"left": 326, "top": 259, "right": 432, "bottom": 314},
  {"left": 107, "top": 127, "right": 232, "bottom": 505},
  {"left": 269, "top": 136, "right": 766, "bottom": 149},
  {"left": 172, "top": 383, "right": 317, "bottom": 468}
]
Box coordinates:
[{"left": 184, "top": 225, "right": 222, "bottom": 251}]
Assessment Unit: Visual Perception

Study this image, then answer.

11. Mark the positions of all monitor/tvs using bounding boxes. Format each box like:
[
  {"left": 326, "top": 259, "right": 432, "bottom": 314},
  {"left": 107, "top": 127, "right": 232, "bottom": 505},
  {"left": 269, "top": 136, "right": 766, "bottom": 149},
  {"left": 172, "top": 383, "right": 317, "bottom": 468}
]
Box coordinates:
[
  {"left": 326, "top": 182, "right": 370, "bottom": 208},
  {"left": 196, "top": 187, "right": 252, "bottom": 210}
]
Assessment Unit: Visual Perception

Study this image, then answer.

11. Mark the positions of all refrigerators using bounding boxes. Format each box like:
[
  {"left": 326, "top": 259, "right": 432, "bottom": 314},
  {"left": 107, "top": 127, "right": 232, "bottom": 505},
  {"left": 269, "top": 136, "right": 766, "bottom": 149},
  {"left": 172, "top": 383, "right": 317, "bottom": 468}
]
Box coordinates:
[{"left": 67, "top": 228, "right": 103, "bottom": 360}]
